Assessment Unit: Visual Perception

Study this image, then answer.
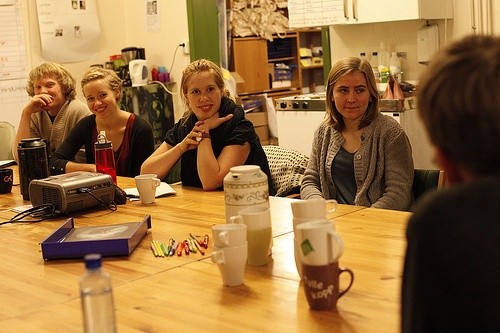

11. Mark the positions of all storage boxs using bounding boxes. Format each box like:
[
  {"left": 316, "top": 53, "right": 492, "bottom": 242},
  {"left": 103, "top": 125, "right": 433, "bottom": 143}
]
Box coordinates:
[{"left": 244, "top": 110, "right": 269, "bottom": 147}]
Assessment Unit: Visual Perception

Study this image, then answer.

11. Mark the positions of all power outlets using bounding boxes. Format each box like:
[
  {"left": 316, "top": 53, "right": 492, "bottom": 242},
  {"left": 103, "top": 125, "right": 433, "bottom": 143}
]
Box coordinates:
[{"left": 181, "top": 41, "right": 189, "bottom": 53}]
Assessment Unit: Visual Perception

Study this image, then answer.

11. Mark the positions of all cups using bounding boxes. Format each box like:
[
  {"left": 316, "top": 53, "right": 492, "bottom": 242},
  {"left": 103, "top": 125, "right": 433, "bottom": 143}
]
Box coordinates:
[
  {"left": 0, "top": 169, "right": 13, "bottom": 194},
  {"left": 135, "top": 174, "right": 161, "bottom": 204},
  {"left": 293, "top": 218, "right": 325, "bottom": 236},
  {"left": 211, "top": 241, "right": 249, "bottom": 287},
  {"left": 230, "top": 206, "right": 273, "bottom": 266},
  {"left": 296, "top": 218, "right": 343, "bottom": 264},
  {"left": 291, "top": 198, "right": 337, "bottom": 217},
  {"left": 211, "top": 222, "right": 246, "bottom": 246},
  {"left": 151, "top": 66, "right": 170, "bottom": 83},
  {"left": 302, "top": 260, "right": 354, "bottom": 311}
]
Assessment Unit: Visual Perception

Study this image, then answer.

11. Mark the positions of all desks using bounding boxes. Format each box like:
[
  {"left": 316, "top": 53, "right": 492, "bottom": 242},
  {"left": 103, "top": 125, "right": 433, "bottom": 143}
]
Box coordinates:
[{"left": 0, "top": 164, "right": 412, "bottom": 333}]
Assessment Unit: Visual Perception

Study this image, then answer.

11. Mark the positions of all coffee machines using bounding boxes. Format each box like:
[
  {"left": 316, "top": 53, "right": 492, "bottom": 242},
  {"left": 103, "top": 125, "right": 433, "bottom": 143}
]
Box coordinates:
[{"left": 122, "top": 47, "right": 146, "bottom": 63}]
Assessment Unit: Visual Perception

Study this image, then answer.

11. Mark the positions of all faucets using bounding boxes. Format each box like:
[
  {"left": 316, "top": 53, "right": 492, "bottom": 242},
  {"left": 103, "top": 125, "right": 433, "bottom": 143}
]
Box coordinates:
[{"left": 386, "top": 71, "right": 394, "bottom": 89}]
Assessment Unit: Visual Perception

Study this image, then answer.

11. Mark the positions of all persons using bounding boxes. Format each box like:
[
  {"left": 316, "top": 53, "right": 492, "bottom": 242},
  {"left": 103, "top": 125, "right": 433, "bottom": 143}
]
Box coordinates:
[
  {"left": 299, "top": 57, "right": 414, "bottom": 212},
  {"left": 47, "top": 66, "right": 154, "bottom": 180},
  {"left": 11, "top": 62, "right": 94, "bottom": 165},
  {"left": 401, "top": 35, "right": 500, "bottom": 333},
  {"left": 140, "top": 59, "right": 277, "bottom": 197}
]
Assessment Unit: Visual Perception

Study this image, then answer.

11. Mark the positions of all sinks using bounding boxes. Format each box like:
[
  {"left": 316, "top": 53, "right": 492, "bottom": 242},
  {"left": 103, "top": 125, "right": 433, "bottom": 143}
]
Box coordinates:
[{"left": 378, "top": 93, "right": 415, "bottom": 112}]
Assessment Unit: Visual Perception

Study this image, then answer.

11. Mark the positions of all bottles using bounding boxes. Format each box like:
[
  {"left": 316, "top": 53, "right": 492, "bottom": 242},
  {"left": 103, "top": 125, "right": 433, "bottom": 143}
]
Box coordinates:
[
  {"left": 390, "top": 51, "right": 401, "bottom": 82},
  {"left": 378, "top": 41, "right": 391, "bottom": 68},
  {"left": 78, "top": 253, "right": 117, "bottom": 333},
  {"left": 90, "top": 55, "right": 127, "bottom": 87},
  {"left": 94, "top": 130, "right": 118, "bottom": 187},
  {"left": 359, "top": 53, "right": 368, "bottom": 60},
  {"left": 369, "top": 52, "right": 379, "bottom": 82}
]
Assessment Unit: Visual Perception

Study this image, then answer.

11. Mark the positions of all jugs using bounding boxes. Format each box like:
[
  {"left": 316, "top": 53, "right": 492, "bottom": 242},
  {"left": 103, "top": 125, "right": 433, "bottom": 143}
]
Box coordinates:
[
  {"left": 118, "top": 64, "right": 131, "bottom": 86},
  {"left": 16, "top": 137, "right": 50, "bottom": 200},
  {"left": 224, "top": 164, "right": 270, "bottom": 223}
]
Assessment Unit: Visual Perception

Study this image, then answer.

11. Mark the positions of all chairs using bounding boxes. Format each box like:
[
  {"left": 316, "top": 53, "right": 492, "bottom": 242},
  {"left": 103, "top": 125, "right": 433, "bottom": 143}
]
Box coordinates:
[
  {"left": 262, "top": 145, "right": 309, "bottom": 199},
  {"left": 411, "top": 169, "right": 447, "bottom": 202}
]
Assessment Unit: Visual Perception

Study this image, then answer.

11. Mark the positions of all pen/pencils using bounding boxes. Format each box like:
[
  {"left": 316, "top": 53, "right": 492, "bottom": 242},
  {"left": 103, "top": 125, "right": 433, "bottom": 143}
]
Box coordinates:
[{"left": 151, "top": 233, "right": 209, "bottom": 258}]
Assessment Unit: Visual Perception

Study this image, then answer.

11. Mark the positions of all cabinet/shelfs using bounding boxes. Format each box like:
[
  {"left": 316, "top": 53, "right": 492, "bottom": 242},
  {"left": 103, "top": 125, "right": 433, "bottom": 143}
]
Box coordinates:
[
  {"left": 230, "top": 31, "right": 302, "bottom": 102},
  {"left": 288, "top": 0, "right": 453, "bottom": 29}
]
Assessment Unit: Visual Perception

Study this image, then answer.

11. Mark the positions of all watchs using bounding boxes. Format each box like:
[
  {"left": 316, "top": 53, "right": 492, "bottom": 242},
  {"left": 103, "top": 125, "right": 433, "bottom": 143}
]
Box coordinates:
[{"left": 195, "top": 132, "right": 211, "bottom": 142}]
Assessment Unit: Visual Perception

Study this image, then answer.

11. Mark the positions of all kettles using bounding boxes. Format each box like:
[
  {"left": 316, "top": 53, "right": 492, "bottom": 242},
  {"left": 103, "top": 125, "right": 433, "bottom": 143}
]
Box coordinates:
[{"left": 128, "top": 59, "right": 150, "bottom": 87}]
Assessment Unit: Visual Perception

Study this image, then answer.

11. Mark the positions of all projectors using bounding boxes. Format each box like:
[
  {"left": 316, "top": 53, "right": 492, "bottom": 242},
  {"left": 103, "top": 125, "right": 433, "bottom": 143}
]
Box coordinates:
[{"left": 29, "top": 170, "right": 115, "bottom": 214}]
